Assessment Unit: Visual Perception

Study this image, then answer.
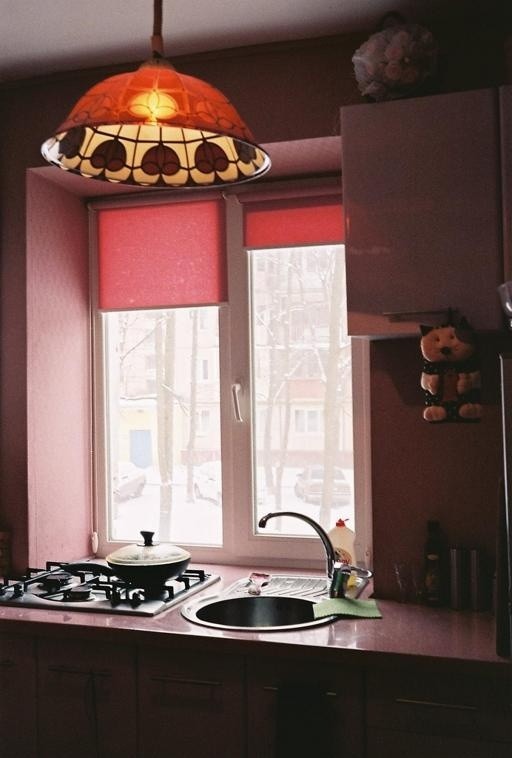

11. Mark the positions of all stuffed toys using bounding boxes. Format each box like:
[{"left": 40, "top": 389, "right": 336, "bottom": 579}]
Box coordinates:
[{"left": 418, "top": 315, "right": 485, "bottom": 424}]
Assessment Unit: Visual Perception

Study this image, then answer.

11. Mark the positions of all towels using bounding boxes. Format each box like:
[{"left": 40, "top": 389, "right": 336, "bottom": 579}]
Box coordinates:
[{"left": 312, "top": 598, "right": 382, "bottom": 618}]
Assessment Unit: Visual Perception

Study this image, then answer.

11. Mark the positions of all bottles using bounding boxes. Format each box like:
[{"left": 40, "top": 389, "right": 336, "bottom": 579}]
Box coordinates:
[{"left": 420, "top": 516, "right": 446, "bottom": 608}]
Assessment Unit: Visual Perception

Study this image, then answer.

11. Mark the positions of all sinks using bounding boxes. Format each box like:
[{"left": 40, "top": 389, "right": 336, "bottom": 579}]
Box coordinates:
[{"left": 181, "top": 596, "right": 338, "bottom": 631}]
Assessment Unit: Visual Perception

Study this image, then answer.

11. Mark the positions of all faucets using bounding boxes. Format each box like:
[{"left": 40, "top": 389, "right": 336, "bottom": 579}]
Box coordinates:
[{"left": 258, "top": 511, "right": 336, "bottom": 597}]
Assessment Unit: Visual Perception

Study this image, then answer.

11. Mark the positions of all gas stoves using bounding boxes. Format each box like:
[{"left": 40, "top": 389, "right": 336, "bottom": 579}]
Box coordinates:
[{"left": 1, "top": 561, "right": 221, "bottom": 618}]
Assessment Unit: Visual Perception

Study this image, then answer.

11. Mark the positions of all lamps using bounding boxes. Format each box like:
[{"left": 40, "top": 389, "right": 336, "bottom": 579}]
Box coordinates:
[{"left": 41, "top": 0, "right": 271, "bottom": 189}]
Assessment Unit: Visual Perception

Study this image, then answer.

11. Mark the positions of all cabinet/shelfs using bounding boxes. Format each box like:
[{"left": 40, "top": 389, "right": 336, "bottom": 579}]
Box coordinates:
[
  {"left": 338, "top": 85, "right": 512, "bottom": 336},
  {"left": 0, "top": 618, "right": 512, "bottom": 758}
]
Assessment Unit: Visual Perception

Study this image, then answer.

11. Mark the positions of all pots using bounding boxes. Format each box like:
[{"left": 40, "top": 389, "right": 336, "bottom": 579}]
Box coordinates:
[{"left": 106, "top": 529, "right": 192, "bottom": 585}]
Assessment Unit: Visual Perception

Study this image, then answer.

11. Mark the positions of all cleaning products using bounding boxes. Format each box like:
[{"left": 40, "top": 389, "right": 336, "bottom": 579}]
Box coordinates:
[{"left": 328, "top": 518, "right": 355, "bottom": 566}]
